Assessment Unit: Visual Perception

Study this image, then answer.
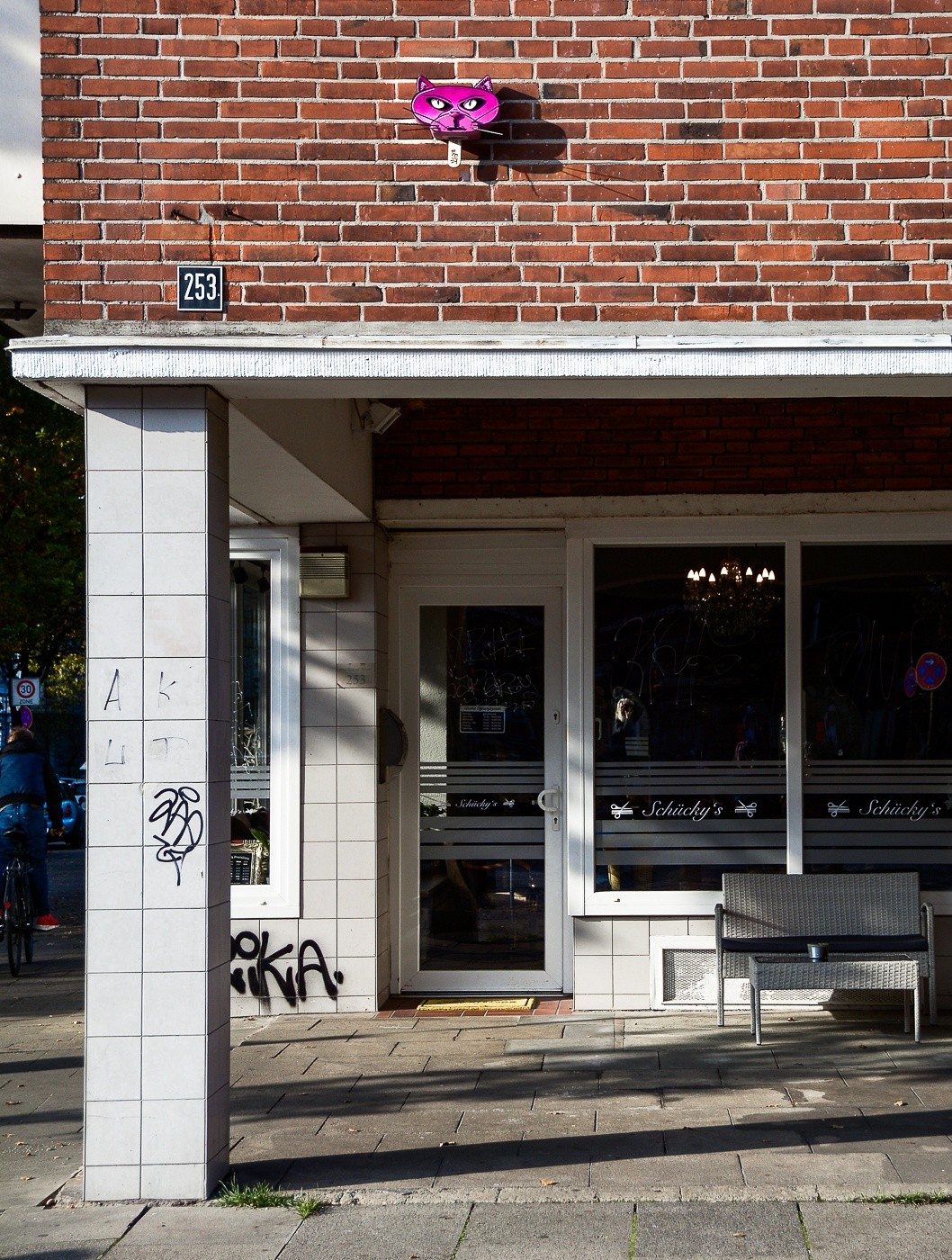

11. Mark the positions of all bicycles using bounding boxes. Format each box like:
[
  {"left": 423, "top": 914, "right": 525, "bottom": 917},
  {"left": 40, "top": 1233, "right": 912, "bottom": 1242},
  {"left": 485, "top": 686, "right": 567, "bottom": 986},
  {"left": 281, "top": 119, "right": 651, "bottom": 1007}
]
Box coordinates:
[{"left": 0, "top": 807, "right": 51, "bottom": 979}]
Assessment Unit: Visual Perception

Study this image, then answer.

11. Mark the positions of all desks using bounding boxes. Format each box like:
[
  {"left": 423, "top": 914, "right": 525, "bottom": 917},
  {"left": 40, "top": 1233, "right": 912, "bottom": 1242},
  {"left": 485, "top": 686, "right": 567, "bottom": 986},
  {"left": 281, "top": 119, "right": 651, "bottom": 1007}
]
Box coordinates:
[{"left": 747, "top": 954, "right": 921, "bottom": 1045}]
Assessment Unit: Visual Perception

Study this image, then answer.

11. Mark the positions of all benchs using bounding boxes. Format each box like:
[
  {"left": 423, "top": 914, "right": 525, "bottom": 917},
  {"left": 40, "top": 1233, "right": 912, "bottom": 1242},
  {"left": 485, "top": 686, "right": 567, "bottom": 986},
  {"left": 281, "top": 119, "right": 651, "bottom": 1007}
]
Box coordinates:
[{"left": 714, "top": 870, "right": 940, "bottom": 1028}]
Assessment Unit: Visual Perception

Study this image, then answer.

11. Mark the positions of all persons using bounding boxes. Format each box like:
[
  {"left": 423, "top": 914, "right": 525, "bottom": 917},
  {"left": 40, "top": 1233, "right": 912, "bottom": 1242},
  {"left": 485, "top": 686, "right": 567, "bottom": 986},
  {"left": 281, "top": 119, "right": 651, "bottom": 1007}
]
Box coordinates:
[
  {"left": 0, "top": 728, "right": 65, "bottom": 932},
  {"left": 615, "top": 699, "right": 635, "bottom": 722}
]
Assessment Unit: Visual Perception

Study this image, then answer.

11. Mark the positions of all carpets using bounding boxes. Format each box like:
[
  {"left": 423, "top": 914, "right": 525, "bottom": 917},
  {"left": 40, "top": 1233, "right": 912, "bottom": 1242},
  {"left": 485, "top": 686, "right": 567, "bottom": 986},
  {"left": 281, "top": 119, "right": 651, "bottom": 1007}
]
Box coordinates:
[{"left": 415, "top": 998, "right": 536, "bottom": 1013}]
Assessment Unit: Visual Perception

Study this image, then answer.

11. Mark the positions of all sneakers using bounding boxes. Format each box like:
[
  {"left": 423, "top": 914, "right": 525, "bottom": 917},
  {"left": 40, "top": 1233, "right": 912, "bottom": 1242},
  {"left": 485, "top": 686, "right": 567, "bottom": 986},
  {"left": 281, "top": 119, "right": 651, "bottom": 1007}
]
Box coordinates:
[
  {"left": 33, "top": 914, "right": 60, "bottom": 931},
  {"left": 0, "top": 909, "right": 4, "bottom": 919}
]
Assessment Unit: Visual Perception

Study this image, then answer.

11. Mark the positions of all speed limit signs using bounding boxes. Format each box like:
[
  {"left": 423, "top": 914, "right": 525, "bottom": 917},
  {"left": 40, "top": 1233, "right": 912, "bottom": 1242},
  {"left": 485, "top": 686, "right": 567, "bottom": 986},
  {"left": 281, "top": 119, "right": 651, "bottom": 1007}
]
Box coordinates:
[{"left": 17, "top": 681, "right": 35, "bottom": 697}]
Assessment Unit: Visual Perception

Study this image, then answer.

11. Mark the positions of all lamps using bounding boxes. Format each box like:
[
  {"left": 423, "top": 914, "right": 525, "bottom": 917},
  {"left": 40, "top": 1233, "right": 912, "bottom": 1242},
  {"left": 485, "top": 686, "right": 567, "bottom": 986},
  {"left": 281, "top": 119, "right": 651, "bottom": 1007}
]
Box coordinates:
[
  {"left": 682, "top": 548, "right": 783, "bottom": 641},
  {"left": 351, "top": 401, "right": 402, "bottom": 435}
]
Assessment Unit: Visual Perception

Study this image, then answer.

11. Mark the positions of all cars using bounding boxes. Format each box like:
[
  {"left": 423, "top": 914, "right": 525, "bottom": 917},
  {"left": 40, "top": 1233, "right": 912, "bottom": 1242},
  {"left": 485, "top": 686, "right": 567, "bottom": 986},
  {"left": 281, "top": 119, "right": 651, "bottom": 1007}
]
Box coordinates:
[
  {"left": 737, "top": 693, "right": 841, "bottom": 756},
  {"left": 42, "top": 777, "right": 87, "bottom": 846},
  {"left": 229, "top": 814, "right": 270, "bottom": 878}
]
